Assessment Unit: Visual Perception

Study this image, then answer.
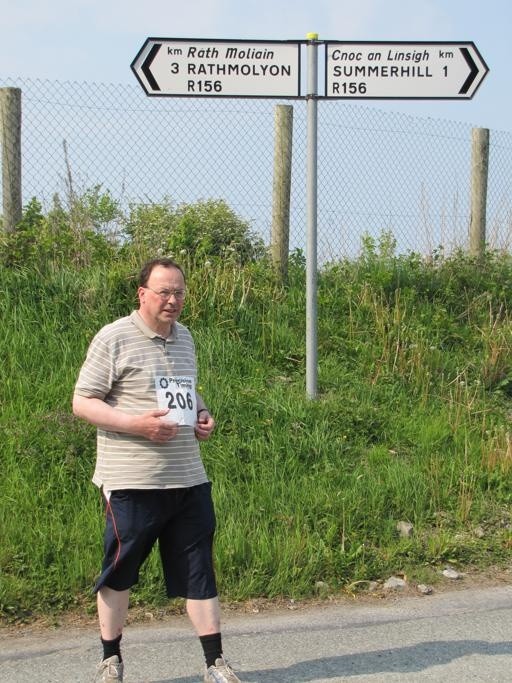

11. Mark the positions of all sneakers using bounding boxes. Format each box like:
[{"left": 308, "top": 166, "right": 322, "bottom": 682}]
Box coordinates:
[
  {"left": 204, "top": 658, "right": 240, "bottom": 682},
  {"left": 96, "top": 655, "right": 125, "bottom": 683}
]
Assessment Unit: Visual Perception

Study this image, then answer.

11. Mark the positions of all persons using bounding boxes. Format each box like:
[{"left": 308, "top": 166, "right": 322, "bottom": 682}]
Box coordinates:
[{"left": 71, "top": 254, "right": 245, "bottom": 683}]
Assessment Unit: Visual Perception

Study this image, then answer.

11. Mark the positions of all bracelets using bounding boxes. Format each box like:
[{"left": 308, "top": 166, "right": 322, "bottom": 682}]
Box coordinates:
[{"left": 197, "top": 408, "right": 209, "bottom": 417}]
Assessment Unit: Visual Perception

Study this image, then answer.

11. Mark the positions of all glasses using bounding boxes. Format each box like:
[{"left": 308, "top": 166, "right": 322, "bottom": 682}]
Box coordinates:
[{"left": 147, "top": 288, "right": 186, "bottom": 302}]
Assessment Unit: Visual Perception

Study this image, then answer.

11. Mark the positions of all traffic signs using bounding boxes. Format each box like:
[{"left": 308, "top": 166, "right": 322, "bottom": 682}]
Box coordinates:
[
  {"left": 129, "top": 38, "right": 301, "bottom": 99},
  {"left": 324, "top": 41, "right": 489, "bottom": 99}
]
